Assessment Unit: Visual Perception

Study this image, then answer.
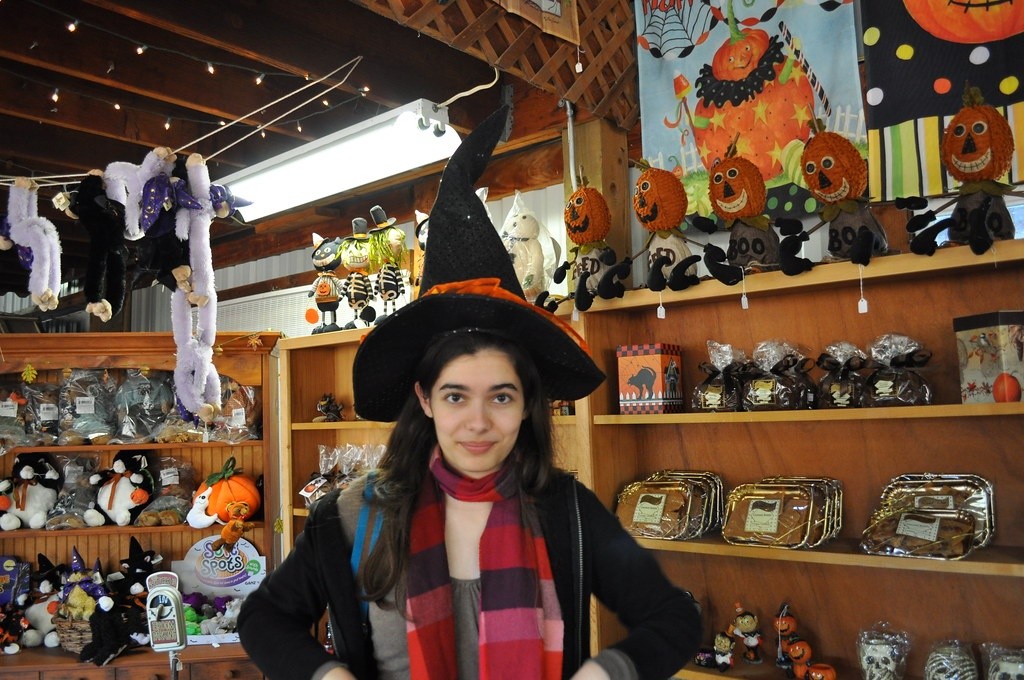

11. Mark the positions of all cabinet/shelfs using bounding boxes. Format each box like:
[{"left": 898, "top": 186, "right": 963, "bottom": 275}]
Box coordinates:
[{"left": 0, "top": 233, "right": 1023, "bottom": 678}]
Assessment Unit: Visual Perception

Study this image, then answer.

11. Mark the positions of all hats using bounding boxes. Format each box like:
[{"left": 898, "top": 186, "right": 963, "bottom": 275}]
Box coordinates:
[{"left": 351, "top": 102, "right": 606, "bottom": 424}]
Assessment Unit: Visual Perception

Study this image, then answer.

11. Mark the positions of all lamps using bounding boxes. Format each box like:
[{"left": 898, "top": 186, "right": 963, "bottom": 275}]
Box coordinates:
[{"left": 210, "top": 98, "right": 464, "bottom": 229}]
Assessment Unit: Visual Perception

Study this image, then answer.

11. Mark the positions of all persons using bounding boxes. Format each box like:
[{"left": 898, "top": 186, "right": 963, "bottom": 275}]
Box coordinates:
[{"left": 234, "top": 328, "right": 704, "bottom": 680}]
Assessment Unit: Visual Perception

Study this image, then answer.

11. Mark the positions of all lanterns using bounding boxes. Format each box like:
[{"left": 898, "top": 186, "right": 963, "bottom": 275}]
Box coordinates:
[
  {"left": 773, "top": 600, "right": 836, "bottom": 680},
  {"left": 628, "top": 158, "right": 689, "bottom": 245},
  {"left": 796, "top": 102, "right": 875, "bottom": 220},
  {"left": 706, "top": 129, "right": 776, "bottom": 233},
  {"left": 563, "top": 164, "right": 613, "bottom": 254},
  {"left": 693, "top": 0, "right": 816, "bottom": 183},
  {"left": 938, "top": 78, "right": 1016, "bottom": 197}
]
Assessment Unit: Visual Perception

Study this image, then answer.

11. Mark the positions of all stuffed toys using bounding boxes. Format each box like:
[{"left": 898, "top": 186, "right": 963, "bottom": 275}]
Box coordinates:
[{"left": 0, "top": 145, "right": 260, "bottom": 668}]
